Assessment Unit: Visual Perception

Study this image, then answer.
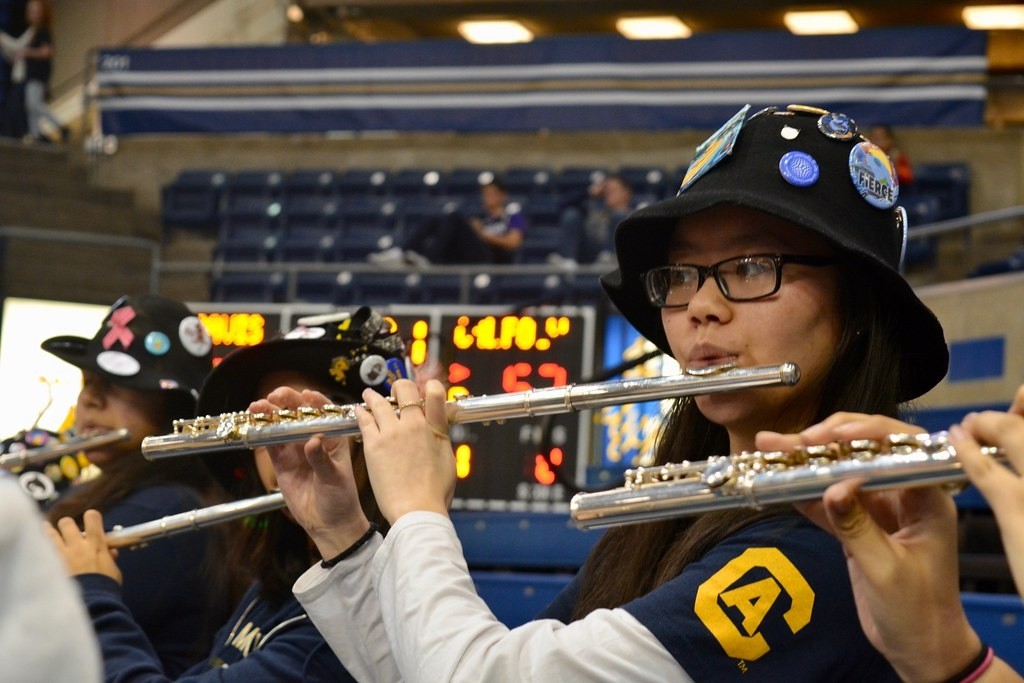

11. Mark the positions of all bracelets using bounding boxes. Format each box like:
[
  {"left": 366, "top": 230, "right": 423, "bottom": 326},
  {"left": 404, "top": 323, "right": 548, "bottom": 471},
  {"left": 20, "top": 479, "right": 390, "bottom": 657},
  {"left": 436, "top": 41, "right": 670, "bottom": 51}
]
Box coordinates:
[{"left": 940, "top": 644, "right": 995, "bottom": 683}]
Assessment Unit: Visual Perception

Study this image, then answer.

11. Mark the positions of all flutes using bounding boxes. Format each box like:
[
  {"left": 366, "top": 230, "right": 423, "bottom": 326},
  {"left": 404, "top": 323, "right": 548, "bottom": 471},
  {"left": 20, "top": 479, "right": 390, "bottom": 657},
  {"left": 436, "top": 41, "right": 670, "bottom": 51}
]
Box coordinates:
[
  {"left": 140, "top": 360, "right": 802, "bottom": 461},
  {"left": 79, "top": 488, "right": 288, "bottom": 552},
  {"left": 0, "top": 427, "right": 131, "bottom": 469},
  {"left": 568, "top": 430, "right": 1009, "bottom": 533}
]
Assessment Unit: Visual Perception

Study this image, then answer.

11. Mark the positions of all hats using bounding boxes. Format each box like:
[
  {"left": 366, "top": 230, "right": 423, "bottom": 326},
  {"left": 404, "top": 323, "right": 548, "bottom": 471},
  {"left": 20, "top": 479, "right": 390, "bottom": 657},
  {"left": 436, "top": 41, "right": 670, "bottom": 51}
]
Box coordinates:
[
  {"left": 41, "top": 294, "right": 212, "bottom": 399},
  {"left": 600, "top": 105, "right": 949, "bottom": 407},
  {"left": 195, "top": 305, "right": 407, "bottom": 498}
]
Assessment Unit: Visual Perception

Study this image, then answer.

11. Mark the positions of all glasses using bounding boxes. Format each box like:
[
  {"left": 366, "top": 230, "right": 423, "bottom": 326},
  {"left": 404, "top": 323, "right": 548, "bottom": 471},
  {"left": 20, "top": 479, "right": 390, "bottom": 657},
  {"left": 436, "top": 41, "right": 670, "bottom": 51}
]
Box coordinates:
[{"left": 640, "top": 253, "right": 838, "bottom": 308}]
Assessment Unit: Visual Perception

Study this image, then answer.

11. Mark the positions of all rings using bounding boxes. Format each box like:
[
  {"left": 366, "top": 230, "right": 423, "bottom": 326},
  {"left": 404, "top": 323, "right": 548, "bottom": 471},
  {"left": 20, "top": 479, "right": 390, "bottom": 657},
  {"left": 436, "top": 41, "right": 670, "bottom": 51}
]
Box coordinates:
[{"left": 397, "top": 401, "right": 424, "bottom": 414}]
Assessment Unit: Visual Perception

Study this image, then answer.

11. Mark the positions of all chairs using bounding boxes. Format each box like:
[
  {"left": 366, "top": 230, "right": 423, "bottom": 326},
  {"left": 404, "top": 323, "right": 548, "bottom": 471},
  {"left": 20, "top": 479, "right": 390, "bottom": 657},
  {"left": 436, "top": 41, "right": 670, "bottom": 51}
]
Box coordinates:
[{"left": 160, "top": 160, "right": 973, "bottom": 308}]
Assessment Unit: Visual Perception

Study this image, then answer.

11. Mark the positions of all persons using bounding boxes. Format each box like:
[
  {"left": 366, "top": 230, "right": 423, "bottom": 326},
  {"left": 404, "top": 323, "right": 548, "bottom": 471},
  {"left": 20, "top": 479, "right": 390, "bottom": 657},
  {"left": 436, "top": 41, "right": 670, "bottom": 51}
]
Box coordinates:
[
  {"left": 1, "top": 291, "right": 410, "bottom": 683},
  {"left": 370, "top": 175, "right": 531, "bottom": 268},
  {"left": 12, "top": 0, "right": 69, "bottom": 145},
  {"left": 555, "top": 175, "right": 635, "bottom": 268},
  {"left": 754, "top": 382, "right": 1024, "bottom": 683},
  {"left": 867, "top": 123, "right": 912, "bottom": 192},
  {"left": 245, "top": 100, "right": 951, "bottom": 683}
]
